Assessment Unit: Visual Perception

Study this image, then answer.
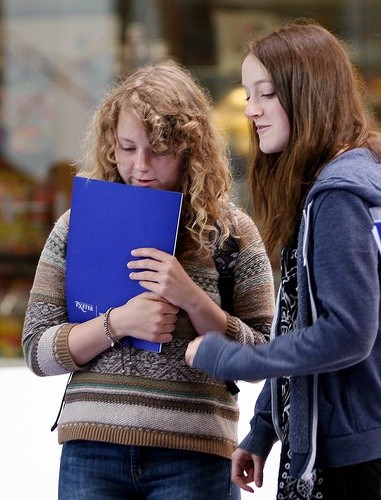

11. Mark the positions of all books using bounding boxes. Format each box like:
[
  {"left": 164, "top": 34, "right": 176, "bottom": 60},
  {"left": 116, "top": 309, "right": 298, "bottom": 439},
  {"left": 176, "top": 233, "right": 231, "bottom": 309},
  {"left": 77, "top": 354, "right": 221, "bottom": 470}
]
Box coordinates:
[{"left": 65, "top": 176, "right": 184, "bottom": 353}]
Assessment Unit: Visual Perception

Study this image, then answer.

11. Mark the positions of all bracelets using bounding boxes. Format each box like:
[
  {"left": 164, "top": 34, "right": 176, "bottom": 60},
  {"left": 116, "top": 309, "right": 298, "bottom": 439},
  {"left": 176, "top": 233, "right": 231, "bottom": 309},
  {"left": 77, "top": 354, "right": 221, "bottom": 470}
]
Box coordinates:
[{"left": 103, "top": 307, "right": 119, "bottom": 347}]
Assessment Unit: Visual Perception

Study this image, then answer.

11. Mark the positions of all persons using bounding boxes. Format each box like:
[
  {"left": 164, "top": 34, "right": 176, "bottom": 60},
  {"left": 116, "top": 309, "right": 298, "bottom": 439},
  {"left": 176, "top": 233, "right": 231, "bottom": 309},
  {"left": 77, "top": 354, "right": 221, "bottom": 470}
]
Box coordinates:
[
  {"left": 21, "top": 64, "right": 276, "bottom": 500},
  {"left": 185, "top": 17, "right": 381, "bottom": 500}
]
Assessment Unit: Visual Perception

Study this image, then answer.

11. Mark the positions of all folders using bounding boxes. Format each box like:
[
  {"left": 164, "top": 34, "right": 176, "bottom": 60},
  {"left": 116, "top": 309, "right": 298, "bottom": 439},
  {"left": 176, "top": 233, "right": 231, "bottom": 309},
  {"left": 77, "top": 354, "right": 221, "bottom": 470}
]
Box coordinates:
[{"left": 63, "top": 176, "right": 184, "bottom": 354}]
